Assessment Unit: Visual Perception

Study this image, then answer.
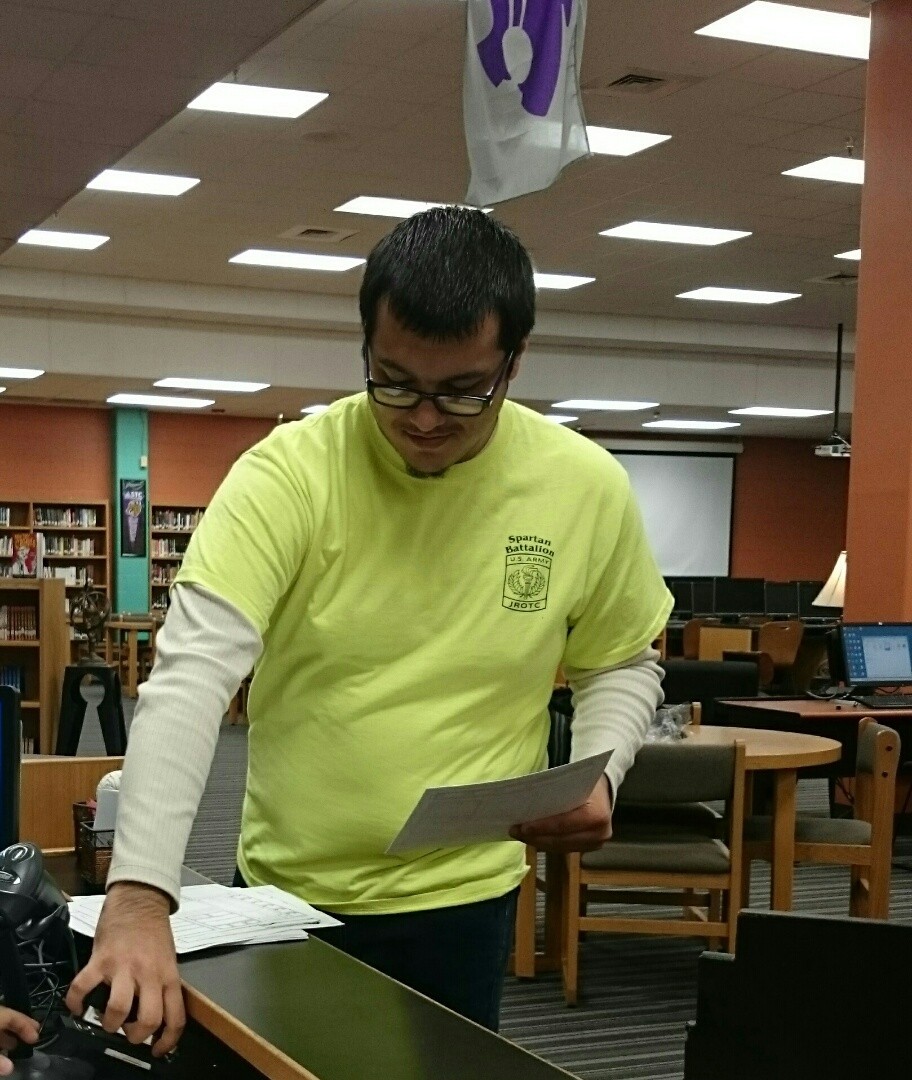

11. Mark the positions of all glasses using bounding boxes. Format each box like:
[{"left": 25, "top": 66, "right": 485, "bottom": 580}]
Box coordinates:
[{"left": 363, "top": 335, "right": 516, "bottom": 417}]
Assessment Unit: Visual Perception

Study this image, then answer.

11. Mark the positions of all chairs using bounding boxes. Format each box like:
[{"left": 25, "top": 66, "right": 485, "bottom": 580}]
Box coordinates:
[{"left": 505, "top": 574, "right": 910, "bottom": 1079}]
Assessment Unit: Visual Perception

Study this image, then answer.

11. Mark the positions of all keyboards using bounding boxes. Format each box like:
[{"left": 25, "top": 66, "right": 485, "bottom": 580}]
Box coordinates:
[{"left": 852, "top": 693, "right": 912, "bottom": 709}]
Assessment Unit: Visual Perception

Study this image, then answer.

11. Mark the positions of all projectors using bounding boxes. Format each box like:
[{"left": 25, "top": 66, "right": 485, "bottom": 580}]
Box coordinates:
[{"left": 814, "top": 442, "right": 851, "bottom": 460}]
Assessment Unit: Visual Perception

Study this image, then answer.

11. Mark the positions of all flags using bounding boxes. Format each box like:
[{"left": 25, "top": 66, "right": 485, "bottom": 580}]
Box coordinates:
[{"left": 460, "top": 1, "right": 593, "bottom": 208}]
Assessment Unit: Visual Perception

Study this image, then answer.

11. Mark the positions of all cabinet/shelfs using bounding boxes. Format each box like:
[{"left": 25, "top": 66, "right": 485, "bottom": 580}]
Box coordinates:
[
  {"left": 0, "top": 573, "right": 69, "bottom": 757},
  {"left": 1, "top": 492, "right": 117, "bottom": 668},
  {"left": 150, "top": 503, "right": 208, "bottom": 610}
]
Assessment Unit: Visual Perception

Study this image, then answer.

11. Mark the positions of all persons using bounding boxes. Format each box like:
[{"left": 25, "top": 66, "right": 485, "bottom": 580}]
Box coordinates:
[
  {"left": 0, "top": 1005, "right": 41, "bottom": 1077},
  {"left": 64, "top": 208, "right": 678, "bottom": 1058}
]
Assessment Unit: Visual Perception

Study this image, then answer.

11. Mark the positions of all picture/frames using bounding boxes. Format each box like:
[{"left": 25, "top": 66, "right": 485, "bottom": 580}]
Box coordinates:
[{"left": 120, "top": 477, "right": 148, "bottom": 558}]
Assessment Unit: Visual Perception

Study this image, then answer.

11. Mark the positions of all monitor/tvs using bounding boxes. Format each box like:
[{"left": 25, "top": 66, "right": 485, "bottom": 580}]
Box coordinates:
[
  {"left": 838, "top": 621, "right": 912, "bottom": 686},
  {"left": 764, "top": 582, "right": 796, "bottom": 617},
  {"left": 691, "top": 580, "right": 713, "bottom": 618},
  {"left": 797, "top": 582, "right": 841, "bottom": 619},
  {"left": 669, "top": 580, "right": 690, "bottom": 613},
  {"left": 712, "top": 577, "right": 763, "bottom": 614}
]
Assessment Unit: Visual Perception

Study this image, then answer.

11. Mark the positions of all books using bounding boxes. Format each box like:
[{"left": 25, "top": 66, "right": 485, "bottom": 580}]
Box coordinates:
[{"left": 1, "top": 503, "right": 206, "bottom": 755}]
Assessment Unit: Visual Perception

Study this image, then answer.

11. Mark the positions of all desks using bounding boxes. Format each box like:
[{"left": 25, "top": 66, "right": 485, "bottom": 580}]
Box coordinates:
[{"left": 108, "top": 607, "right": 173, "bottom": 691}]
